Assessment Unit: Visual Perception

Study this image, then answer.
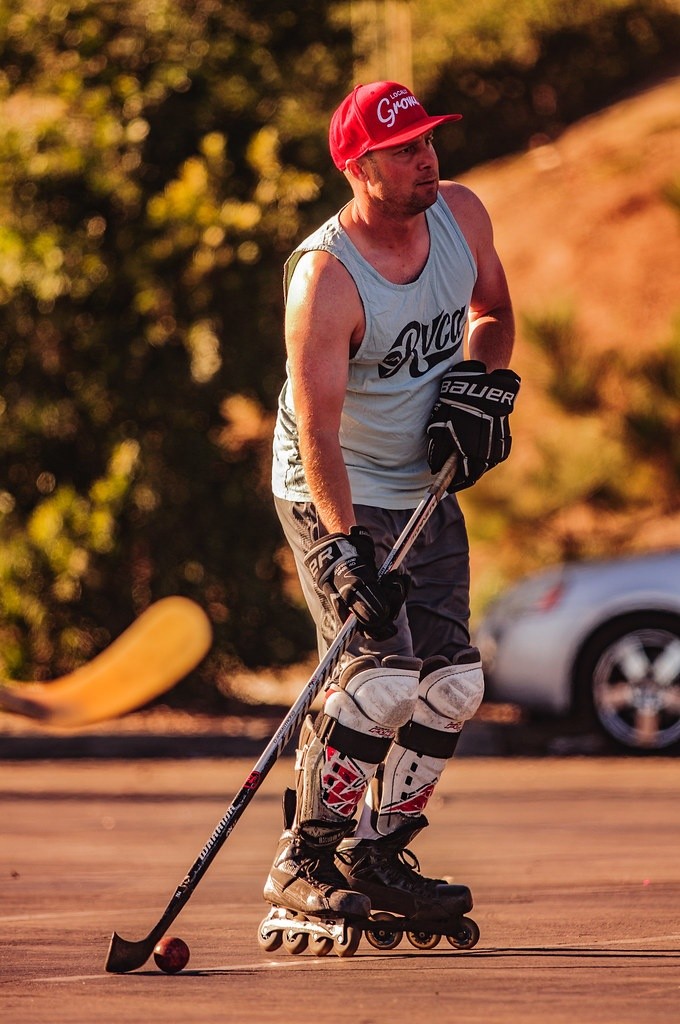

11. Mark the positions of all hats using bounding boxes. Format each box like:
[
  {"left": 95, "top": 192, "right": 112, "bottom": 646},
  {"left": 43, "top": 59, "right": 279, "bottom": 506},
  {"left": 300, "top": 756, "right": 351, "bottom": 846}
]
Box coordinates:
[{"left": 329, "top": 79, "right": 462, "bottom": 172}]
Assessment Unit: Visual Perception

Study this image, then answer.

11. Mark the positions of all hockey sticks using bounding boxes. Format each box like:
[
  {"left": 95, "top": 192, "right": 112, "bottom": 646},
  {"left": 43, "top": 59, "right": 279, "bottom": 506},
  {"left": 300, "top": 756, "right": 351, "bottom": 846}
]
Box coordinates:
[{"left": 103, "top": 452, "right": 460, "bottom": 974}]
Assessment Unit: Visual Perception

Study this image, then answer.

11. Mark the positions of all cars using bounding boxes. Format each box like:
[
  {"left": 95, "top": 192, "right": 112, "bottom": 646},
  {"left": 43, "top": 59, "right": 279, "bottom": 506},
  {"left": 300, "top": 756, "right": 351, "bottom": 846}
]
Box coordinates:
[{"left": 470, "top": 552, "right": 679, "bottom": 756}]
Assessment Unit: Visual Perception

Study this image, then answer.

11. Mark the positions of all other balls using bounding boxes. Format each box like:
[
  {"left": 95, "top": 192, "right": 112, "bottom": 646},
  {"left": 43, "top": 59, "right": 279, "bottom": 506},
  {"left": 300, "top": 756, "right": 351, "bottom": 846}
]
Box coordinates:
[{"left": 153, "top": 937, "right": 191, "bottom": 974}]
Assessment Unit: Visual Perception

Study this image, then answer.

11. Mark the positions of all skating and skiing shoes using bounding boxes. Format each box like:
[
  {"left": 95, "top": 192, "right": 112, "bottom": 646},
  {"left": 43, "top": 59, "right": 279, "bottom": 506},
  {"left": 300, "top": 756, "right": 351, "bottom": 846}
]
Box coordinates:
[
  {"left": 257, "top": 788, "right": 371, "bottom": 958},
  {"left": 331, "top": 815, "right": 479, "bottom": 948}
]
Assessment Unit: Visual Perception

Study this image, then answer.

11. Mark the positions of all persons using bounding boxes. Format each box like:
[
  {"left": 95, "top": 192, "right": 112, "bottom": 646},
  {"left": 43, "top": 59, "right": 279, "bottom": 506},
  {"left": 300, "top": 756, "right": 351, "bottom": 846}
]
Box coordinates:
[{"left": 259, "top": 79, "right": 521, "bottom": 957}]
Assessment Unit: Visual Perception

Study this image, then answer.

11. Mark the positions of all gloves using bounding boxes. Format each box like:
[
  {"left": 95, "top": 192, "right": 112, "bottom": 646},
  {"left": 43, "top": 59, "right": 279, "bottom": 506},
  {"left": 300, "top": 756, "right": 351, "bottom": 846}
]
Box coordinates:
[
  {"left": 301, "top": 527, "right": 411, "bottom": 642},
  {"left": 425, "top": 360, "right": 520, "bottom": 492}
]
